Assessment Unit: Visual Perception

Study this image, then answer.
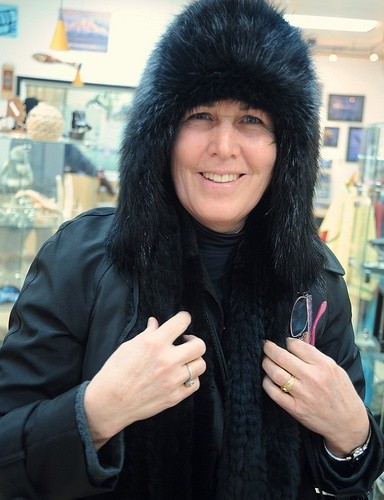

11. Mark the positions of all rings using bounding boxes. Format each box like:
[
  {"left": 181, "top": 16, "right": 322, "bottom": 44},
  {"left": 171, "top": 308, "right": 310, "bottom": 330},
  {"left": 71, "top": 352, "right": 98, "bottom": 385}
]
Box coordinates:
[
  {"left": 184, "top": 364, "right": 194, "bottom": 388},
  {"left": 281, "top": 375, "right": 295, "bottom": 393}
]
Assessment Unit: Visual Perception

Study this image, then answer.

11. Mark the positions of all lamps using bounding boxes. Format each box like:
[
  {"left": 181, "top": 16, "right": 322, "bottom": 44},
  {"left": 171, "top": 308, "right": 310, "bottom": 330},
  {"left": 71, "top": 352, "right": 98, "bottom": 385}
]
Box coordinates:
[
  {"left": 49, "top": 0, "right": 67, "bottom": 51},
  {"left": 71, "top": 64, "right": 84, "bottom": 87}
]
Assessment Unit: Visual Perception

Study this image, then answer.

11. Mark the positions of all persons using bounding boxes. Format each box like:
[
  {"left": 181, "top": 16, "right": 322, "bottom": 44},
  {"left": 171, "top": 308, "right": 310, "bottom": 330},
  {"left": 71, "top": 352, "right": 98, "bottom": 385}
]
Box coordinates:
[
  {"left": 0, "top": 97, "right": 104, "bottom": 274},
  {"left": 0, "top": 0, "right": 384, "bottom": 500}
]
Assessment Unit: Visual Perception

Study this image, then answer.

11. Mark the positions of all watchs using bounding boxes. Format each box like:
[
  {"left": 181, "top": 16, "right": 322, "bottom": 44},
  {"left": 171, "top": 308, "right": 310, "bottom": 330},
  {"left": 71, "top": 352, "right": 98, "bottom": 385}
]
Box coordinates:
[{"left": 323, "top": 426, "right": 372, "bottom": 461}]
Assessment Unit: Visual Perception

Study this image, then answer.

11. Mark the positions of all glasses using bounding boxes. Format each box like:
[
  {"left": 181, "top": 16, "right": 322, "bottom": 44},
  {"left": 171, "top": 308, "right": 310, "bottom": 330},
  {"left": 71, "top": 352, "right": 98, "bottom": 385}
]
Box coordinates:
[{"left": 290, "top": 292, "right": 327, "bottom": 347}]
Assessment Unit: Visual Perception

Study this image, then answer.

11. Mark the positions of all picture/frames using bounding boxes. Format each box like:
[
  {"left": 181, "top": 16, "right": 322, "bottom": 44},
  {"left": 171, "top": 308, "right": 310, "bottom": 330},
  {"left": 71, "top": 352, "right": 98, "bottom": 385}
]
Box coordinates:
[
  {"left": 348, "top": 127, "right": 362, "bottom": 161},
  {"left": 324, "top": 127, "right": 338, "bottom": 146},
  {"left": 328, "top": 94, "right": 364, "bottom": 121}
]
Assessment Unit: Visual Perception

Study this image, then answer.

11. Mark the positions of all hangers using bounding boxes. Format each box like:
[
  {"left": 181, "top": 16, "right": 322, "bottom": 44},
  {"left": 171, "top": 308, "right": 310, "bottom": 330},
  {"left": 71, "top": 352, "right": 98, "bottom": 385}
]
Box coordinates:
[{"left": 344, "top": 170, "right": 384, "bottom": 205}]
